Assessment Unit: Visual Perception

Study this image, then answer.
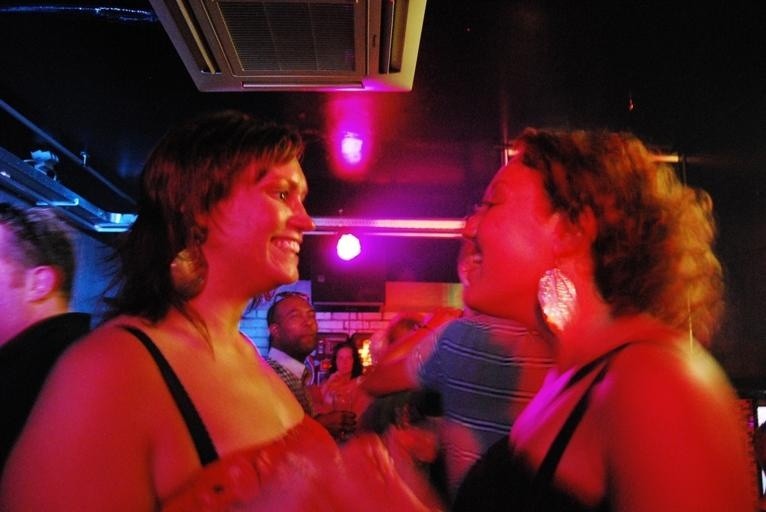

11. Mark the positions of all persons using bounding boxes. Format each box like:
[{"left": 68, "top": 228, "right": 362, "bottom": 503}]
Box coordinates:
[
  {"left": 451, "top": 127, "right": 766, "bottom": 511},
  {"left": 1, "top": 112, "right": 425, "bottom": 512},
  {"left": 0, "top": 202, "right": 94, "bottom": 472}
]
